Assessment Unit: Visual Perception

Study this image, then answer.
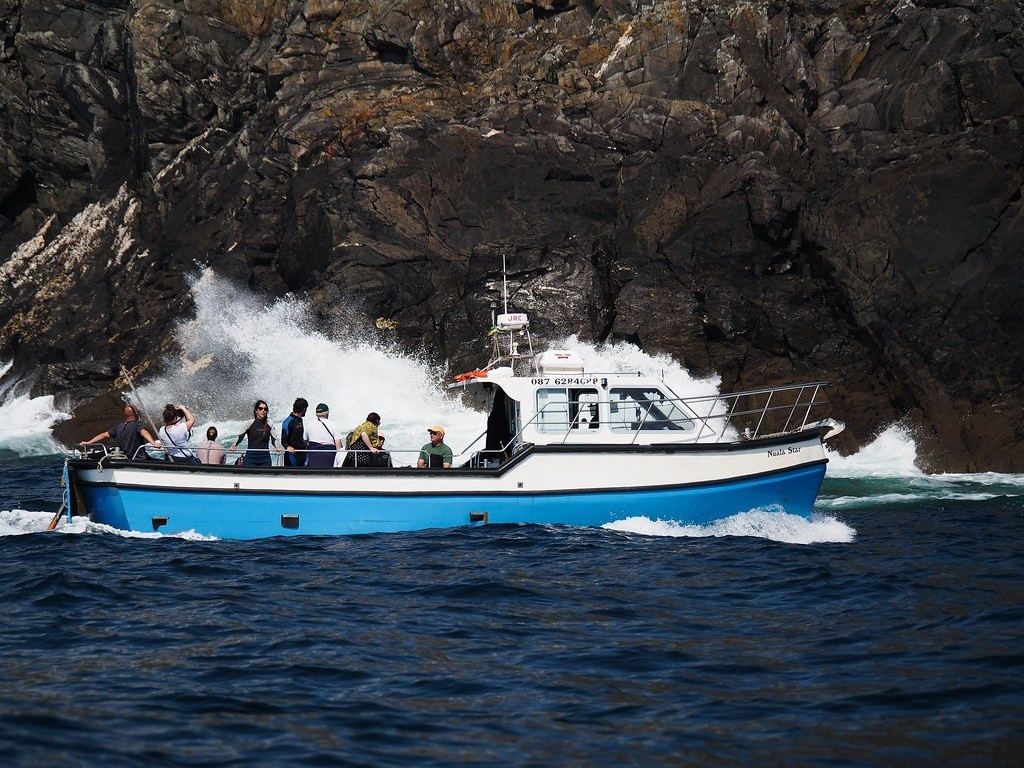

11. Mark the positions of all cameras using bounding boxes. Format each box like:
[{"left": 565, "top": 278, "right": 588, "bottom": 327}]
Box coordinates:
[{"left": 177, "top": 409, "right": 186, "bottom": 417}]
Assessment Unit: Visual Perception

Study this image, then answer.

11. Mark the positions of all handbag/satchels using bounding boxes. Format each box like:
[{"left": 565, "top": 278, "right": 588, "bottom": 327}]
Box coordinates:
[{"left": 193, "top": 456, "right": 202, "bottom": 464}]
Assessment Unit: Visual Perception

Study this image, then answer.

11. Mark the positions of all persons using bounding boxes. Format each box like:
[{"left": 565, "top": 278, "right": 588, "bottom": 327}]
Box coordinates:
[
  {"left": 342, "top": 413, "right": 382, "bottom": 467},
  {"left": 377, "top": 435, "right": 393, "bottom": 467},
  {"left": 416, "top": 426, "right": 452, "bottom": 468},
  {"left": 80, "top": 405, "right": 162, "bottom": 459},
  {"left": 333, "top": 431, "right": 353, "bottom": 467},
  {"left": 196, "top": 427, "right": 225, "bottom": 464},
  {"left": 229, "top": 400, "right": 283, "bottom": 467},
  {"left": 303, "top": 403, "right": 343, "bottom": 468},
  {"left": 158, "top": 404, "right": 195, "bottom": 463},
  {"left": 281, "top": 398, "right": 309, "bottom": 467}
]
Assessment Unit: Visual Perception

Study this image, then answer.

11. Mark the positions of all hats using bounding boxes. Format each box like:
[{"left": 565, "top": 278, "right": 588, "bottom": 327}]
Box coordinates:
[
  {"left": 427, "top": 426, "right": 445, "bottom": 436},
  {"left": 378, "top": 431, "right": 385, "bottom": 439},
  {"left": 316, "top": 403, "right": 329, "bottom": 413}
]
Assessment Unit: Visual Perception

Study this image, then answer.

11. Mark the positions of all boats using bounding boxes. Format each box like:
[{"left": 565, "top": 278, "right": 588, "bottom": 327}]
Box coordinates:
[{"left": 46, "top": 252, "right": 833, "bottom": 532}]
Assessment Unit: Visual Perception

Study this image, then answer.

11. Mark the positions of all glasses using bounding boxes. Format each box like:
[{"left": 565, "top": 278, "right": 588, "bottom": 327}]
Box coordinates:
[
  {"left": 430, "top": 432, "right": 440, "bottom": 435},
  {"left": 258, "top": 407, "right": 268, "bottom": 411},
  {"left": 134, "top": 410, "right": 141, "bottom": 413}
]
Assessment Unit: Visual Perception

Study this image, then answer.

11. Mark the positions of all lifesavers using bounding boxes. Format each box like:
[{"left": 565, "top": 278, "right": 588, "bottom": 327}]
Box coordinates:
[{"left": 453, "top": 366, "right": 514, "bottom": 382}]
